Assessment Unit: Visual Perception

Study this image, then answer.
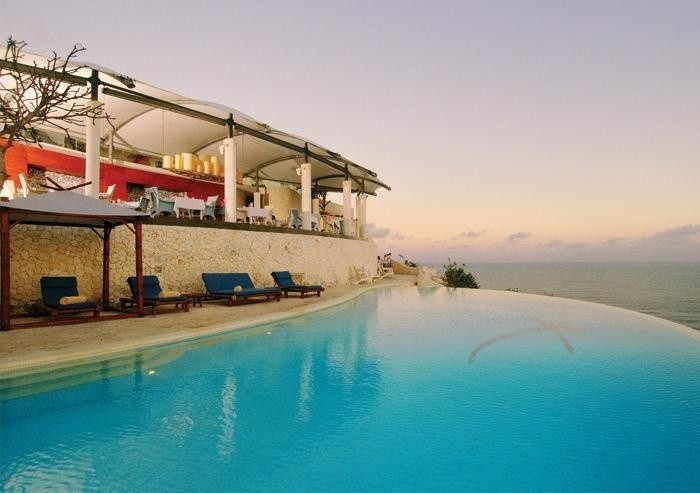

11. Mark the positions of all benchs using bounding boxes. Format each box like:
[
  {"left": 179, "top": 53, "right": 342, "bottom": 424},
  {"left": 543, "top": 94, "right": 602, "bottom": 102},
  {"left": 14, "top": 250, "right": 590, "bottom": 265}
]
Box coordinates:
[{"left": 201, "top": 272, "right": 282, "bottom": 306}]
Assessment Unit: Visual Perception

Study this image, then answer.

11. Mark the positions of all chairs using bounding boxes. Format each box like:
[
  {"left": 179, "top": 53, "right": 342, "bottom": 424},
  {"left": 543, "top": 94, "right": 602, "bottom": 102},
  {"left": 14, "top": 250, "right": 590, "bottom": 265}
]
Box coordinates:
[
  {"left": 271, "top": 271, "right": 324, "bottom": 298},
  {"left": 139, "top": 186, "right": 187, "bottom": 218},
  {"left": 1, "top": 172, "right": 40, "bottom": 201},
  {"left": 99, "top": 183, "right": 116, "bottom": 203},
  {"left": 120, "top": 276, "right": 189, "bottom": 317},
  {"left": 351, "top": 264, "right": 393, "bottom": 286},
  {"left": 312, "top": 213, "right": 344, "bottom": 233},
  {"left": 288, "top": 209, "right": 302, "bottom": 229},
  {"left": 201, "top": 195, "right": 225, "bottom": 222},
  {"left": 32, "top": 276, "right": 98, "bottom": 323}
]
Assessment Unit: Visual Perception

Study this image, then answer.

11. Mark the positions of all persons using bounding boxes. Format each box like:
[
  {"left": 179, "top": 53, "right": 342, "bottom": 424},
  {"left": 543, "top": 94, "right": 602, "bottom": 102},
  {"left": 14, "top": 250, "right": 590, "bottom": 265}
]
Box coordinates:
[{"left": 378, "top": 252, "right": 392, "bottom": 267}]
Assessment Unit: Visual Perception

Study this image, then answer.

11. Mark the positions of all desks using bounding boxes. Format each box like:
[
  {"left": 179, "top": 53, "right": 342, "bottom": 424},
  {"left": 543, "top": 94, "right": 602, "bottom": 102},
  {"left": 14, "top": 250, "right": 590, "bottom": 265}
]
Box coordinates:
[
  {"left": 173, "top": 197, "right": 205, "bottom": 220},
  {"left": 175, "top": 292, "right": 206, "bottom": 312},
  {"left": 237, "top": 206, "right": 268, "bottom": 225}
]
